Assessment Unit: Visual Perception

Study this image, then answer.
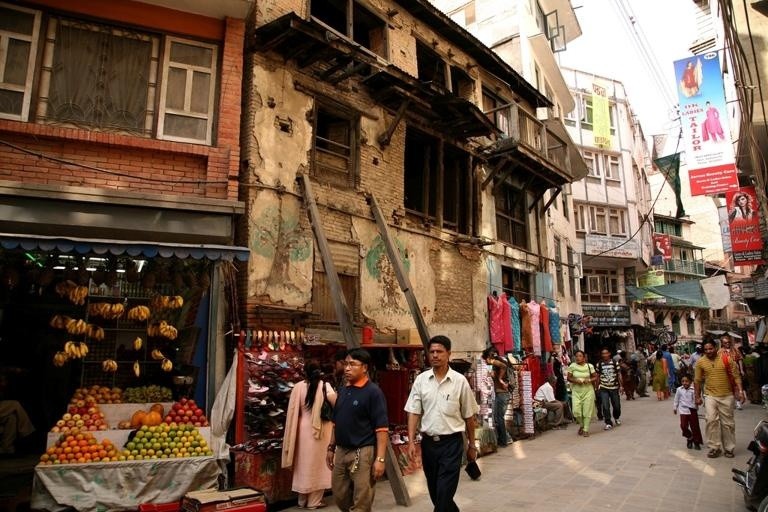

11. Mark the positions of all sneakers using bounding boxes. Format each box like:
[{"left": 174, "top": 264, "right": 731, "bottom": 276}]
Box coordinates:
[
  {"left": 724, "top": 449, "right": 734, "bottom": 457},
  {"left": 687, "top": 439, "right": 702, "bottom": 451},
  {"left": 706, "top": 448, "right": 722, "bottom": 458},
  {"left": 578, "top": 429, "right": 589, "bottom": 437},
  {"left": 615, "top": 416, "right": 622, "bottom": 425},
  {"left": 603, "top": 424, "right": 613, "bottom": 430}
]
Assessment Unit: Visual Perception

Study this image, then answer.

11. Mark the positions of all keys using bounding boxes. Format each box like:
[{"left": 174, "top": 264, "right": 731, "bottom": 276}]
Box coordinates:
[{"left": 351, "top": 447, "right": 360, "bottom": 473}]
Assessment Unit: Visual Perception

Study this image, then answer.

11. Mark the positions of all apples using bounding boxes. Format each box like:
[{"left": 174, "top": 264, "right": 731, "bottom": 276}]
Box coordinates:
[
  {"left": 160, "top": 397, "right": 210, "bottom": 426},
  {"left": 51, "top": 395, "right": 109, "bottom": 430}
]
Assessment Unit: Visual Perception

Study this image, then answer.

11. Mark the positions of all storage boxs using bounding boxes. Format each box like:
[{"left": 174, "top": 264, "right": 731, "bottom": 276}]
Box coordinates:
[
  {"left": 396, "top": 328, "right": 423, "bottom": 345},
  {"left": 180, "top": 487, "right": 267, "bottom": 512}
]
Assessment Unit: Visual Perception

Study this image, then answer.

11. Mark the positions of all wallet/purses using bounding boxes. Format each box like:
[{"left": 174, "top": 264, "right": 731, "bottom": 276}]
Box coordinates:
[{"left": 464, "top": 457, "right": 482, "bottom": 482}]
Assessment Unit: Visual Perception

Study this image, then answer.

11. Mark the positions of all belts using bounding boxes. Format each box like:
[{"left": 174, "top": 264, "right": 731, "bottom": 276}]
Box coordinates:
[{"left": 423, "top": 432, "right": 462, "bottom": 442}]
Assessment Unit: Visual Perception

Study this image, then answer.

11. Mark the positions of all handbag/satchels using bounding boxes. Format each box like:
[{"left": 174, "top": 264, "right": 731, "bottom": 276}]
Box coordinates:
[
  {"left": 320, "top": 398, "right": 335, "bottom": 421},
  {"left": 732, "top": 381, "right": 748, "bottom": 403}
]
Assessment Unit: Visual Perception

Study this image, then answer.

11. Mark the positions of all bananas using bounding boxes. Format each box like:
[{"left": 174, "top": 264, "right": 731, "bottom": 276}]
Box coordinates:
[{"left": 50, "top": 285, "right": 184, "bottom": 376}]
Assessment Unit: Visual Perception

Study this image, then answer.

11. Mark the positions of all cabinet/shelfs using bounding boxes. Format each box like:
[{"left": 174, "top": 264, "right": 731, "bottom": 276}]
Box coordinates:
[
  {"left": 30, "top": 400, "right": 231, "bottom": 512},
  {"left": 80, "top": 277, "right": 173, "bottom": 386}
]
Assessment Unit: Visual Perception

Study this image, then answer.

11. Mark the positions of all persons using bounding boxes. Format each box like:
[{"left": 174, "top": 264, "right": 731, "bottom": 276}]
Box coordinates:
[
  {"left": 534, "top": 335, "right": 768, "bottom": 458},
  {"left": 486, "top": 290, "right": 564, "bottom": 355},
  {"left": 729, "top": 192, "right": 756, "bottom": 221},
  {"left": 654, "top": 240, "right": 665, "bottom": 255},
  {"left": 682, "top": 61, "right": 699, "bottom": 96},
  {"left": 704, "top": 102, "right": 724, "bottom": 140},
  {"left": 403, "top": 336, "right": 480, "bottom": 512},
  {"left": 326, "top": 349, "right": 388, "bottom": 512},
  {"left": 482, "top": 350, "right": 513, "bottom": 447},
  {"left": 283, "top": 360, "right": 338, "bottom": 511},
  {"left": 730, "top": 283, "right": 743, "bottom": 299}
]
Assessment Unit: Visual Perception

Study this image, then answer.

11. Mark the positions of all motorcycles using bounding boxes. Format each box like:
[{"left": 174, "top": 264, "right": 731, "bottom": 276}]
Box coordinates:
[{"left": 730, "top": 420, "right": 767, "bottom": 511}]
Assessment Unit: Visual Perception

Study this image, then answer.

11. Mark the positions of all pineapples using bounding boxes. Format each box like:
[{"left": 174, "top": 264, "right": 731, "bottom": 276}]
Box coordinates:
[{"left": 4, "top": 255, "right": 211, "bottom": 291}]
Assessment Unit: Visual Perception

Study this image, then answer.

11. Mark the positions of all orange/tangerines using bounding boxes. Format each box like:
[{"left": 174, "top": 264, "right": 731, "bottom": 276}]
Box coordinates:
[
  {"left": 71, "top": 385, "right": 122, "bottom": 403},
  {"left": 40, "top": 429, "right": 117, "bottom": 464}
]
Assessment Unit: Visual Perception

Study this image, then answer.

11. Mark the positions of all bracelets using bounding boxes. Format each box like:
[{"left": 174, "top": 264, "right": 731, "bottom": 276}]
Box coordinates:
[
  {"left": 468, "top": 445, "right": 478, "bottom": 450},
  {"left": 327, "top": 444, "right": 336, "bottom": 452},
  {"left": 376, "top": 456, "right": 385, "bottom": 462}
]
